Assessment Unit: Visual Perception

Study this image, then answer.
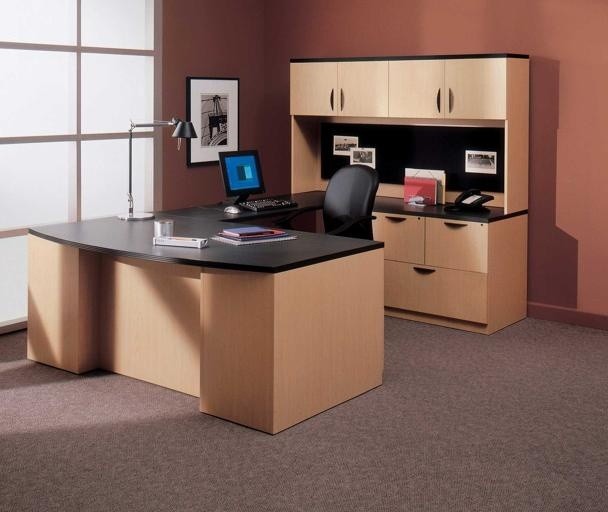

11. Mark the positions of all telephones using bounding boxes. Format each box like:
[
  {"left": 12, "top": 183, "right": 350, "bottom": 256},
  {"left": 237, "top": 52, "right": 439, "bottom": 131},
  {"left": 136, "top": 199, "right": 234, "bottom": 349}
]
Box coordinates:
[{"left": 455, "top": 188, "right": 494, "bottom": 211}]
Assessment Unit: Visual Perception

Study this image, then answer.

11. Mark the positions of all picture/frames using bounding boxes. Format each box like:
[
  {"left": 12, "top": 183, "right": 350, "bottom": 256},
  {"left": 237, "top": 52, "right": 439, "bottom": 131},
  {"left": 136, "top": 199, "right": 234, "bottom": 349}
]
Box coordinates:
[{"left": 186, "top": 75, "right": 241, "bottom": 168}]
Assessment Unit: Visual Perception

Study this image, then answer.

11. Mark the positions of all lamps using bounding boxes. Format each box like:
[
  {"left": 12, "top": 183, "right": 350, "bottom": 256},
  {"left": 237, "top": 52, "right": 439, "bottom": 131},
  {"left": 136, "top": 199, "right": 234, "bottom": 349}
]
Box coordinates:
[{"left": 117, "top": 116, "right": 198, "bottom": 221}]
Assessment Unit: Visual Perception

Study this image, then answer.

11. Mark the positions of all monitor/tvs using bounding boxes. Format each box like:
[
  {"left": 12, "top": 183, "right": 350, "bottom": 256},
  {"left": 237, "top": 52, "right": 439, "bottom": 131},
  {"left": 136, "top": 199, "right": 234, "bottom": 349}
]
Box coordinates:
[{"left": 218, "top": 150, "right": 266, "bottom": 204}]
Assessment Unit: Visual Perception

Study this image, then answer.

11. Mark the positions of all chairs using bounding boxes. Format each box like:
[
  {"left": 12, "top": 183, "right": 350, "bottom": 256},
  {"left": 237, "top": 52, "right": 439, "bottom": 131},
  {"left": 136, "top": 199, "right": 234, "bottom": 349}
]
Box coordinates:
[{"left": 271, "top": 164, "right": 381, "bottom": 240}]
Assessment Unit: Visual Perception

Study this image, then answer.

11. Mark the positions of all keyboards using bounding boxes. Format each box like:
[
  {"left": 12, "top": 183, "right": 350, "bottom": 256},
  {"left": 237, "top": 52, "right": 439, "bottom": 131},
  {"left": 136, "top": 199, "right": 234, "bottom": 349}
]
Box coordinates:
[{"left": 239, "top": 198, "right": 298, "bottom": 212}]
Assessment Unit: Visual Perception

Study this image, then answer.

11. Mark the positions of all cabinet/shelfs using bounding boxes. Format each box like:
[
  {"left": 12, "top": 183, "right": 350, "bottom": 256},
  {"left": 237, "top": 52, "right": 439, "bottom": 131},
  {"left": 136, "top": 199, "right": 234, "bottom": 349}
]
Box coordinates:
[
  {"left": 386, "top": 53, "right": 531, "bottom": 120},
  {"left": 287, "top": 57, "right": 387, "bottom": 117}
]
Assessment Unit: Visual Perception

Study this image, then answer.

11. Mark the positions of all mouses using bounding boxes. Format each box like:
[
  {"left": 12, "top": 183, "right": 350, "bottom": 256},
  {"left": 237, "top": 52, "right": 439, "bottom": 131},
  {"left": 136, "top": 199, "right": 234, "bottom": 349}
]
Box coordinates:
[{"left": 224, "top": 206, "right": 242, "bottom": 214}]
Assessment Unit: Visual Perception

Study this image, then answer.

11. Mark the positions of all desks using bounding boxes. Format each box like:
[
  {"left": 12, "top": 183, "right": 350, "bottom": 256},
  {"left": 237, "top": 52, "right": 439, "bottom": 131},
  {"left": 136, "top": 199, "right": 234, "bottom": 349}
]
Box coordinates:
[{"left": 24, "top": 190, "right": 532, "bottom": 437}]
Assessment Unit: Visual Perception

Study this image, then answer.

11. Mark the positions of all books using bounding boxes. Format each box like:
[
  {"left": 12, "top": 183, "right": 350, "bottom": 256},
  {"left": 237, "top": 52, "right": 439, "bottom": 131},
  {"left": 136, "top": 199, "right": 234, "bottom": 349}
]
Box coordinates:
[
  {"left": 211, "top": 226, "right": 297, "bottom": 245},
  {"left": 403, "top": 168, "right": 446, "bottom": 205}
]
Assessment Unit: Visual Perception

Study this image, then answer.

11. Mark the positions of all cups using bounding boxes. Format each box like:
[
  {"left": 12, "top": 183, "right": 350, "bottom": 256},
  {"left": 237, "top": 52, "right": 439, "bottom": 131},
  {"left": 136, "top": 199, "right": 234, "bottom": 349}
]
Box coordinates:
[{"left": 153, "top": 220, "right": 173, "bottom": 238}]
